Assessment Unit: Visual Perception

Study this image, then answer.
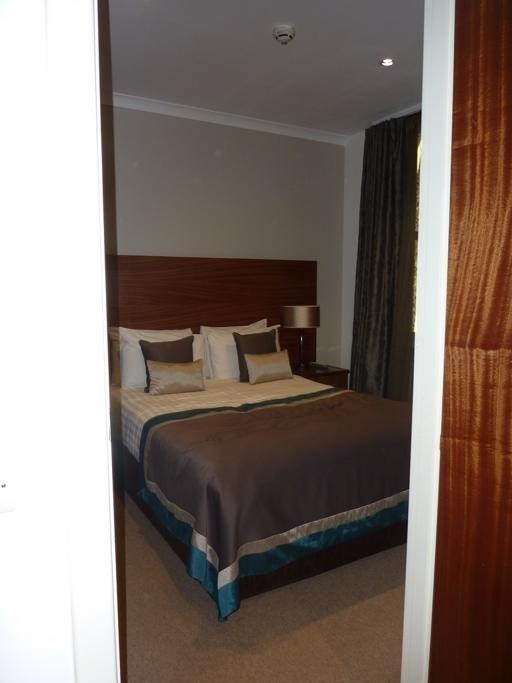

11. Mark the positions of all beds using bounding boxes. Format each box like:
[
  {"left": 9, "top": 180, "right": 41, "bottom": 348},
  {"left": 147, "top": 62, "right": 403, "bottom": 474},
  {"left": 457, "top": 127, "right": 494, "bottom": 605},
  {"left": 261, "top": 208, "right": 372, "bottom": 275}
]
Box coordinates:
[{"left": 108, "top": 254, "right": 412, "bottom": 624}]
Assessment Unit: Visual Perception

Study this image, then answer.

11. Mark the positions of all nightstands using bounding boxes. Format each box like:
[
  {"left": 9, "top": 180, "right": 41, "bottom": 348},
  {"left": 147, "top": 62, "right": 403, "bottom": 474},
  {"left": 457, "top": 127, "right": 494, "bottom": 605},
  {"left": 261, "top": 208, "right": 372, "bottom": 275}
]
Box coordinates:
[{"left": 310, "top": 365, "right": 350, "bottom": 389}]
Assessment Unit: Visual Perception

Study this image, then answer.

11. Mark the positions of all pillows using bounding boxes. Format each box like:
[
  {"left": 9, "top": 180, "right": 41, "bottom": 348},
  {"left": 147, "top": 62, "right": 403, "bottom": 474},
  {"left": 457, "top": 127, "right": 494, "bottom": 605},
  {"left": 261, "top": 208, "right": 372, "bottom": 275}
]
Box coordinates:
[{"left": 108, "top": 319, "right": 294, "bottom": 395}]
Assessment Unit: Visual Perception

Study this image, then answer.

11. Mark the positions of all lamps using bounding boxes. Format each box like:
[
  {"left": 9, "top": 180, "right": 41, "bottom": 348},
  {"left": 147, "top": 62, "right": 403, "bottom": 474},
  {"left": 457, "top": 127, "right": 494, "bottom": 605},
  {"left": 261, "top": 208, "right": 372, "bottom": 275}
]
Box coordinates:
[{"left": 283, "top": 305, "right": 320, "bottom": 373}]
